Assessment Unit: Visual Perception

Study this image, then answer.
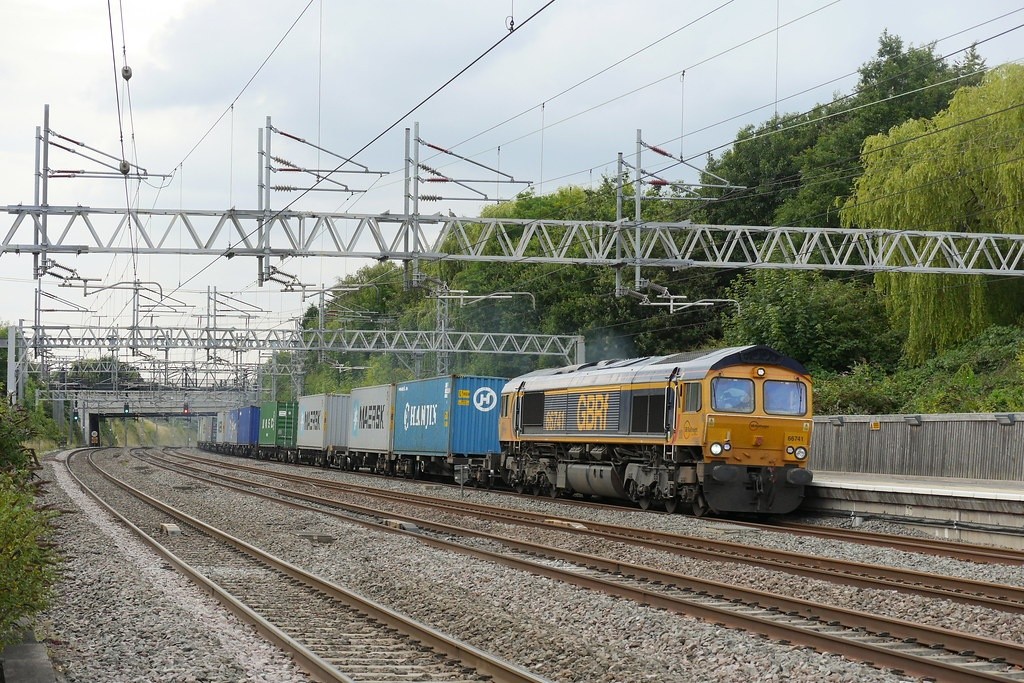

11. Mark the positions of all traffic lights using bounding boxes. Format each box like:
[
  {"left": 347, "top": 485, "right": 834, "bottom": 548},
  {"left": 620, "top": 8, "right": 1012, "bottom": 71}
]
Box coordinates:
[
  {"left": 73, "top": 411, "right": 78, "bottom": 422},
  {"left": 183, "top": 404, "right": 189, "bottom": 415},
  {"left": 124, "top": 404, "right": 130, "bottom": 414}
]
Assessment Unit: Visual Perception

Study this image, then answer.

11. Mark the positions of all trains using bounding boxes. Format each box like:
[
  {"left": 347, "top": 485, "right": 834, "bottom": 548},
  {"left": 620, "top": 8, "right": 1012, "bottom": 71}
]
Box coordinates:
[{"left": 196, "top": 344, "right": 815, "bottom": 518}]
